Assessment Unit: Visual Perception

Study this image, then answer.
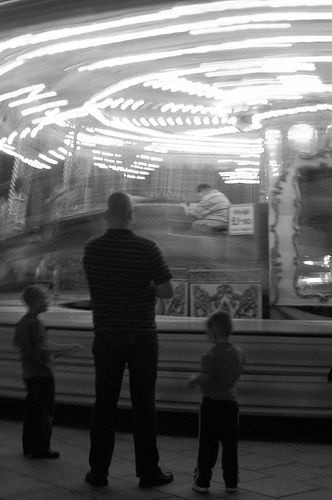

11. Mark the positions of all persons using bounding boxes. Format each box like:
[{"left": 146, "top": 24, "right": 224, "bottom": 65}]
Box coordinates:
[
  {"left": 179, "top": 182, "right": 232, "bottom": 235},
  {"left": 81, "top": 191, "right": 175, "bottom": 489},
  {"left": 11, "top": 284, "right": 83, "bottom": 461},
  {"left": 184, "top": 310, "right": 248, "bottom": 493}
]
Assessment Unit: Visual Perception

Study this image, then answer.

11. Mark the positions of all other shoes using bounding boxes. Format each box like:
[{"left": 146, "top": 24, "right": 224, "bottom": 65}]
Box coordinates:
[
  {"left": 85, "top": 470, "right": 108, "bottom": 488},
  {"left": 23, "top": 449, "right": 35, "bottom": 455},
  {"left": 32, "top": 452, "right": 60, "bottom": 459},
  {"left": 225, "top": 484, "right": 238, "bottom": 492},
  {"left": 192, "top": 481, "right": 209, "bottom": 493},
  {"left": 138, "top": 471, "right": 174, "bottom": 488}
]
